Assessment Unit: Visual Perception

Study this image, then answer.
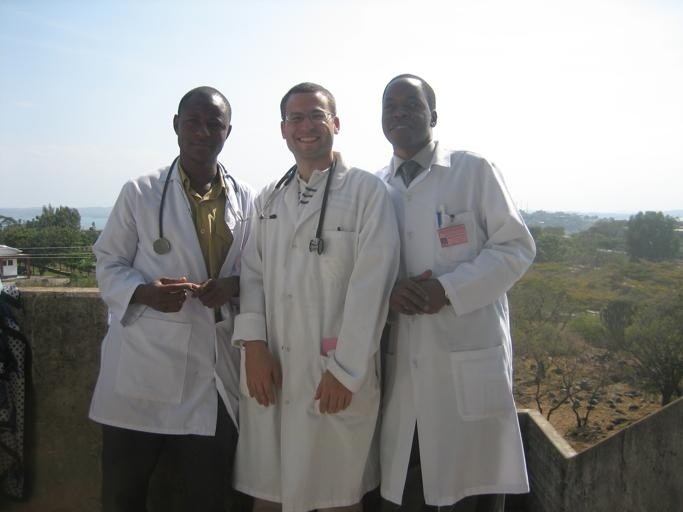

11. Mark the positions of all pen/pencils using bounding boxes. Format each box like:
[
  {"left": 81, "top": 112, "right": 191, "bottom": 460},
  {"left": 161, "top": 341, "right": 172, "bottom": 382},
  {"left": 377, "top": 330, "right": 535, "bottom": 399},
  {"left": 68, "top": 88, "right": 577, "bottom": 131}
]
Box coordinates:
[{"left": 436, "top": 203, "right": 447, "bottom": 229}]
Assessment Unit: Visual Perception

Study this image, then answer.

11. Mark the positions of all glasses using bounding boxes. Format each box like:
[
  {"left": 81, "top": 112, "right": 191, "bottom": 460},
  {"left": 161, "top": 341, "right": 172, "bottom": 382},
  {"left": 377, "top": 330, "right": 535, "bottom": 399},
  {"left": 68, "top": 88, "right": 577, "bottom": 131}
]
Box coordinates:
[{"left": 283, "top": 109, "right": 333, "bottom": 127}]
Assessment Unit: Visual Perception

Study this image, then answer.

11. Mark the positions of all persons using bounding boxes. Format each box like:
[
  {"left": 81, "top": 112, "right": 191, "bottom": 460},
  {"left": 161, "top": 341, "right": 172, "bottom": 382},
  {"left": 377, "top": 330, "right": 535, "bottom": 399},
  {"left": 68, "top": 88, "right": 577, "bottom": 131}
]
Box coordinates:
[
  {"left": 230, "top": 83, "right": 399, "bottom": 511},
  {"left": 86, "top": 85, "right": 254, "bottom": 511},
  {"left": 374, "top": 74, "right": 537, "bottom": 510}
]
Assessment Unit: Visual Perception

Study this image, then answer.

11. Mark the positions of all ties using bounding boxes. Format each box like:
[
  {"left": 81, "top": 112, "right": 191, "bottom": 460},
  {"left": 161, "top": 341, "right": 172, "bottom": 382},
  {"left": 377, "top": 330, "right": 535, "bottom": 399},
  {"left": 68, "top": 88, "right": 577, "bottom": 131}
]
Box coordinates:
[{"left": 400, "top": 160, "right": 418, "bottom": 188}]
